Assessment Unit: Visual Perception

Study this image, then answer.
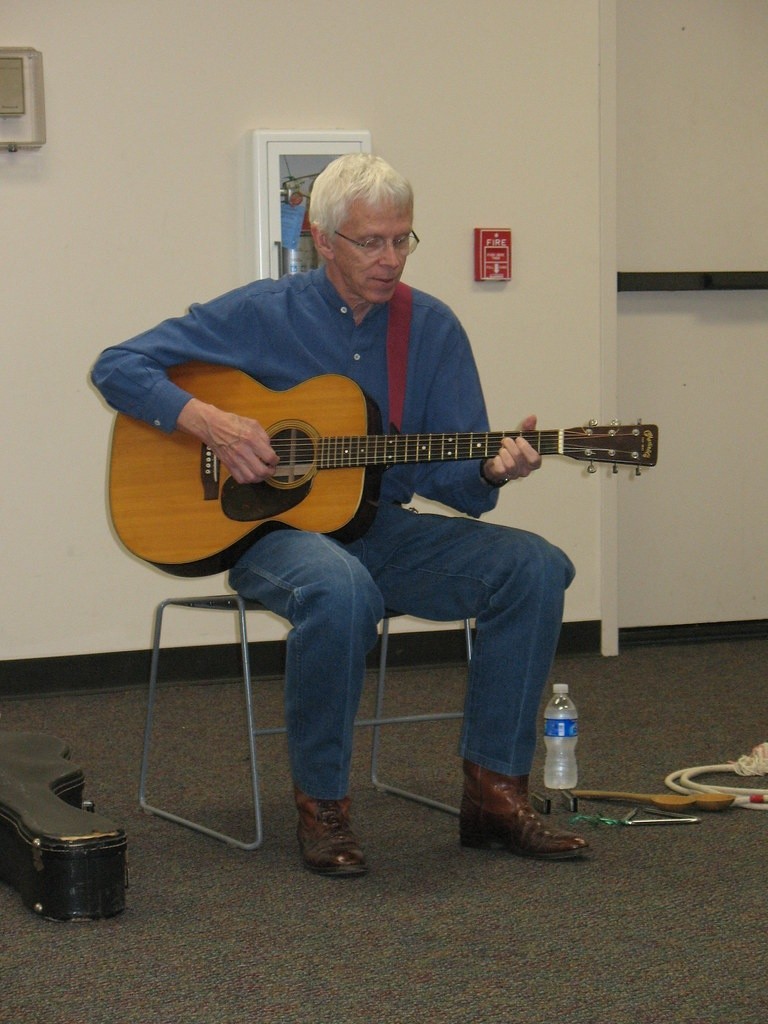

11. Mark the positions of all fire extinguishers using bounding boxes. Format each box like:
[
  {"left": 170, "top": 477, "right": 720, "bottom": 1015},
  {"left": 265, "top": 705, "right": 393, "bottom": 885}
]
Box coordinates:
[{"left": 280, "top": 175, "right": 320, "bottom": 275}]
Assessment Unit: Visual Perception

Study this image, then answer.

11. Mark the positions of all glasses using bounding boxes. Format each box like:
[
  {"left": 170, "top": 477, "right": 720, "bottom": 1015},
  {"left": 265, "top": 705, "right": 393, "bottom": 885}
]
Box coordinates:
[{"left": 334, "top": 229, "right": 420, "bottom": 258}]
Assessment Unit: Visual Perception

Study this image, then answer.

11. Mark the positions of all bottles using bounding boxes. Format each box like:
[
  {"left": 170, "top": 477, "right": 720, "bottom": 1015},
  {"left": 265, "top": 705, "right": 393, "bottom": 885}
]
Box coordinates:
[{"left": 542, "top": 683, "right": 579, "bottom": 790}]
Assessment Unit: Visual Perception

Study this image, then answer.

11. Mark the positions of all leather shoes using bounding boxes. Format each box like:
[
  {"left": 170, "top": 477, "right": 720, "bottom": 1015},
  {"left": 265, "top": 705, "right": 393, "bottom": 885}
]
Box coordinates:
[
  {"left": 294, "top": 787, "right": 371, "bottom": 876},
  {"left": 459, "top": 759, "right": 595, "bottom": 861}
]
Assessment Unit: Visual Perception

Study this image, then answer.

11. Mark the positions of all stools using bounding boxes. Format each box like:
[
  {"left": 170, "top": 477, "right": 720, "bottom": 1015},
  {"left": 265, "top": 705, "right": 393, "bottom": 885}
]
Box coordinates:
[{"left": 137, "top": 591, "right": 473, "bottom": 852}]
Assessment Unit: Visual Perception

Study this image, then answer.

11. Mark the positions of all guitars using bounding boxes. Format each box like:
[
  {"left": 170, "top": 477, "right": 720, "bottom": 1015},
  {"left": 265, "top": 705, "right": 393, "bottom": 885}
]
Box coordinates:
[{"left": 104, "top": 357, "right": 660, "bottom": 577}]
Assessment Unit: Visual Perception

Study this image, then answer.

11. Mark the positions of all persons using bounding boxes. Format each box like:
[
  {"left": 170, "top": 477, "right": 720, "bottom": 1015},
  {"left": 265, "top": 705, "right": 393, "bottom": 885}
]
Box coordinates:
[{"left": 86, "top": 156, "right": 591, "bottom": 879}]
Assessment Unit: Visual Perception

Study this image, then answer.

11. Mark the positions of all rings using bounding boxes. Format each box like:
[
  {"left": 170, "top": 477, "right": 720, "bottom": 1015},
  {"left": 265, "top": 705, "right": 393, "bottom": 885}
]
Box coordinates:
[{"left": 504, "top": 475, "right": 510, "bottom": 482}]
